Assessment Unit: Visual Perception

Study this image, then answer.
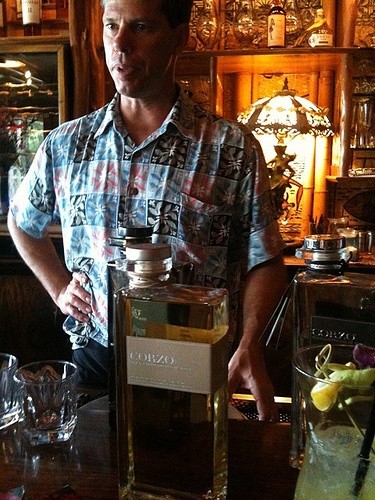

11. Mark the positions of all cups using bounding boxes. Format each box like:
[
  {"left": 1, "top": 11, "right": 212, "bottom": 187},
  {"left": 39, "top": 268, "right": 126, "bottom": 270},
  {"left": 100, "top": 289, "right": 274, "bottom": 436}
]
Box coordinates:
[
  {"left": 290, "top": 342, "right": 375, "bottom": 500},
  {"left": 0, "top": 353, "right": 22, "bottom": 431},
  {"left": 327, "top": 217, "right": 372, "bottom": 261},
  {"left": 11, "top": 359, "right": 79, "bottom": 446}
]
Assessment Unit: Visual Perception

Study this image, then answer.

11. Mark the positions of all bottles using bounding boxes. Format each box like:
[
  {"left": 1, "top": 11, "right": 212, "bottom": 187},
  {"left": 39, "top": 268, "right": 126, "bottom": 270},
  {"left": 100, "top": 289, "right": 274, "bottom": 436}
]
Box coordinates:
[
  {"left": 110, "top": 241, "right": 231, "bottom": 500},
  {"left": 0, "top": 0, "right": 7, "bottom": 37},
  {"left": 22, "top": 0, "right": 42, "bottom": 37},
  {"left": 8, "top": 127, "right": 34, "bottom": 206},
  {"left": 307, "top": 8, "right": 333, "bottom": 48},
  {"left": 267, "top": 0, "right": 287, "bottom": 48}
]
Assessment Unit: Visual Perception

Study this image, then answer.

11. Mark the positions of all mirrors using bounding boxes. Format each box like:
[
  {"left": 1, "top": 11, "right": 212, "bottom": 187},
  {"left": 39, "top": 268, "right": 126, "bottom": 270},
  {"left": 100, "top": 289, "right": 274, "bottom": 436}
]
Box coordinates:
[{"left": 0, "top": 43, "right": 65, "bottom": 238}]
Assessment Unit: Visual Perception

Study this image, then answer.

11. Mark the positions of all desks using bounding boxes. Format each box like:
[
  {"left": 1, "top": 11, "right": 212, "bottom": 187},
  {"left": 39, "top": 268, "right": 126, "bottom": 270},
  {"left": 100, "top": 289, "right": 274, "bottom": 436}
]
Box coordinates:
[
  {"left": 293, "top": 270, "right": 375, "bottom": 351},
  {"left": 0, "top": 408, "right": 297, "bottom": 500}
]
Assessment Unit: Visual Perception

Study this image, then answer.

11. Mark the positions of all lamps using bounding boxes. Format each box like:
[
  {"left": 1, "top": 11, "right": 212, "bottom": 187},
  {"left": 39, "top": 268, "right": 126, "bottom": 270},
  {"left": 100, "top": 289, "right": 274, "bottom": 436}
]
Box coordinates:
[{"left": 234, "top": 76, "right": 338, "bottom": 219}]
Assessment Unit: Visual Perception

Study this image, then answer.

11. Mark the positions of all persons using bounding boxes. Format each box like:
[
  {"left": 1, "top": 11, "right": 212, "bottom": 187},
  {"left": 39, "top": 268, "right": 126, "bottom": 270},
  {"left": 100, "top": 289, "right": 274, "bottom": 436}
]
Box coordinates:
[{"left": 7, "top": 0, "right": 287, "bottom": 423}]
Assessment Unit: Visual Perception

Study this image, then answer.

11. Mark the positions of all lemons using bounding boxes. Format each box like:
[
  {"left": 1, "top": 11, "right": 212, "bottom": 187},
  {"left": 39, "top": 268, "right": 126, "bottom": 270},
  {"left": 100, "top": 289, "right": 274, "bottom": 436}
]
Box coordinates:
[{"left": 310, "top": 379, "right": 342, "bottom": 412}]
